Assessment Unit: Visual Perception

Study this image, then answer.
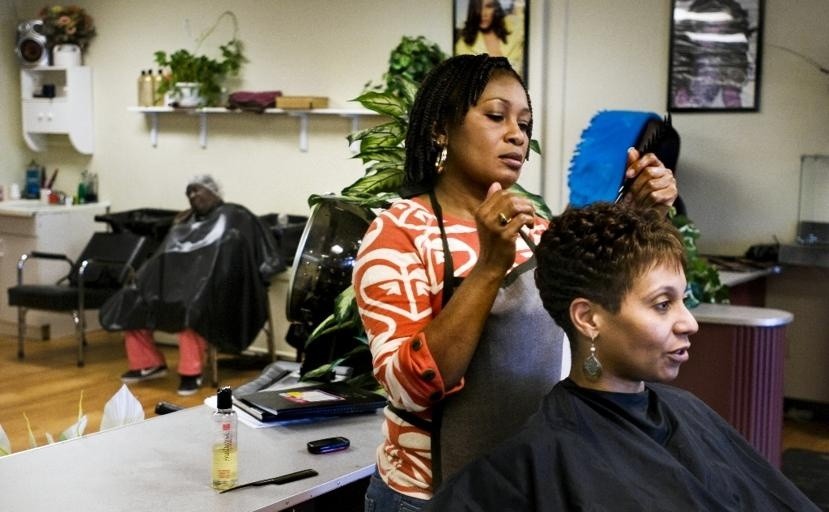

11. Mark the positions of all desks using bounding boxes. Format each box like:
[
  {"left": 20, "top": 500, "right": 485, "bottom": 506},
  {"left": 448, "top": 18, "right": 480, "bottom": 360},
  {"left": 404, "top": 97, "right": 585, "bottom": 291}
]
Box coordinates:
[
  {"left": 2, "top": 202, "right": 106, "bottom": 342},
  {"left": 1, "top": 376, "right": 387, "bottom": 512}
]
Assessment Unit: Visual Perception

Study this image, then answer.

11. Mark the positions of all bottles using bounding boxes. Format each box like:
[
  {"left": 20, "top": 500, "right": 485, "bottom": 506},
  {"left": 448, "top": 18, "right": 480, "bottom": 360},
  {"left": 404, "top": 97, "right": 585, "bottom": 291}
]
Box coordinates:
[
  {"left": 138, "top": 69, "right": 163, "bottom": 107},
  {"left": 211, "top": 388, "right": 238, "bottom": 489}
]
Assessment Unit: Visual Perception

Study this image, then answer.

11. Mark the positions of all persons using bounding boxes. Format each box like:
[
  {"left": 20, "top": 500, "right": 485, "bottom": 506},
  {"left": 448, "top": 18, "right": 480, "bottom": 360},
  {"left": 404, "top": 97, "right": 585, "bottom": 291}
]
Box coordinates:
[
  {"left": 349, "top": 51, "right": 680, "bottom": 512},
  {"left": 116, "top": 172, "right": 257, "bottom": 396},
  {"left": 456, "top": 1, "right": 527, "bottom": 84},
  {"left": 673, "top": 0, "right": 750, "bottom": 108},
  {"left": 428, "top": 198, "right": 824, "bottom": 512}
]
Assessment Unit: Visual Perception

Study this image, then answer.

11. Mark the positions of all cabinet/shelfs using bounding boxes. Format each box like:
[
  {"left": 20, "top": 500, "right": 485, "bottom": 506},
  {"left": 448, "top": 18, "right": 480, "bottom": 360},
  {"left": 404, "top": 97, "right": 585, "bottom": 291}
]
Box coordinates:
[{"left": 20, "top": 64, "right": 94, "bottom": 157}]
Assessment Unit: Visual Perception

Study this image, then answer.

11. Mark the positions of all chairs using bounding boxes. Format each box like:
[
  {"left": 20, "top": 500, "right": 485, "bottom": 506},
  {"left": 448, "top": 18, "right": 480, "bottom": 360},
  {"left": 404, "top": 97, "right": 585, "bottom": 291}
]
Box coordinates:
[
  {"left": 207, "top": 291, "right": 274, "bottom": 384},
  {"left": 7, "top": 231, "right": 146, "bottom": 371}
]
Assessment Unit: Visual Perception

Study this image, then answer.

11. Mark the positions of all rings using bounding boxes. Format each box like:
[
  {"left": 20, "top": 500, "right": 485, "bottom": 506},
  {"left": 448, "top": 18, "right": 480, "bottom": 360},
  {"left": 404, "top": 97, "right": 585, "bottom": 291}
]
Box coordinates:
[{"left": 499, "top": 212, "right": 508, "bottom": 225}]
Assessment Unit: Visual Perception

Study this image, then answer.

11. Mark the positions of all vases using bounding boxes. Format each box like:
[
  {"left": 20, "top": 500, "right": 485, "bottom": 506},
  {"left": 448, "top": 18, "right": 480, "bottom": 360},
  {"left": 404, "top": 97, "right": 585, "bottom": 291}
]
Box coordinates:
[{"left": 46, "top": 40, "right": 88, "bottom": 67}]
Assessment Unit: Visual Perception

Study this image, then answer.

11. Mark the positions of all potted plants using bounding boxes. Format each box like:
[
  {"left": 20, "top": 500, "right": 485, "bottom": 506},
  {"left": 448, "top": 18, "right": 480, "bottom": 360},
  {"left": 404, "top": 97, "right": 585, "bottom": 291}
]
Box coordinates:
[{"left": 154, "top": 8, "right": 248, "bottom": 108}]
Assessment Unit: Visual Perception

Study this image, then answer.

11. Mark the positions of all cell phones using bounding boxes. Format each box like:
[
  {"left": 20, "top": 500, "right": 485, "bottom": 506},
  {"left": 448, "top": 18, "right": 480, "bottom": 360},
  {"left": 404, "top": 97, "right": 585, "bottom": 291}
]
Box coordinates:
[{"left": 307, "top": 436, "right": 350, "bottom": 455}]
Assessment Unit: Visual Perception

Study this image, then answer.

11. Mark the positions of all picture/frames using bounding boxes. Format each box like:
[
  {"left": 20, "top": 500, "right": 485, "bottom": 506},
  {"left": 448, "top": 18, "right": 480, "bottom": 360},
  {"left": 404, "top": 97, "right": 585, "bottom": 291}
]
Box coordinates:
[
  {"left": 667, "top": 0, "right": 765, "bottom": 113},
  {"left": 453, "top": 1, "right": 529, "bottom": 92}
]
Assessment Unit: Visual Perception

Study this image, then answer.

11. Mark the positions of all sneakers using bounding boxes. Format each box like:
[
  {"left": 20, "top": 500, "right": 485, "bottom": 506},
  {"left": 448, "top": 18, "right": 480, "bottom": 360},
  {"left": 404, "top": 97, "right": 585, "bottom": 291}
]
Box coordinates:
[
  {"left": 177, "top": 373, "right": 203, "bottom": 396},
  {"left": 120, "top": 365, "right": 169, "bottom": 383}
]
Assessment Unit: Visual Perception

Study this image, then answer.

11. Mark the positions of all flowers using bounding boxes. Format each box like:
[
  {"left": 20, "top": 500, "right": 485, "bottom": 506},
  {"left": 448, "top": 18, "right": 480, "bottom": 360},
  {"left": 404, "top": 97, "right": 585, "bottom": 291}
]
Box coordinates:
[{"left": 38, "top": 3, "right": 101, "bottom": 54}]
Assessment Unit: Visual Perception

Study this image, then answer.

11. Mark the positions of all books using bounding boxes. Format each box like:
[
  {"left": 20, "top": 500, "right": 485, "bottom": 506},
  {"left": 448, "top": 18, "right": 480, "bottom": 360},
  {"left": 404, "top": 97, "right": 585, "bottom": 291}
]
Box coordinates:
[
  {"left": 274, "top": 93, "right": 329, "bottom": 110},
  {"left": 201, "top": 379, "right": 389, "bottom": 431}
]
